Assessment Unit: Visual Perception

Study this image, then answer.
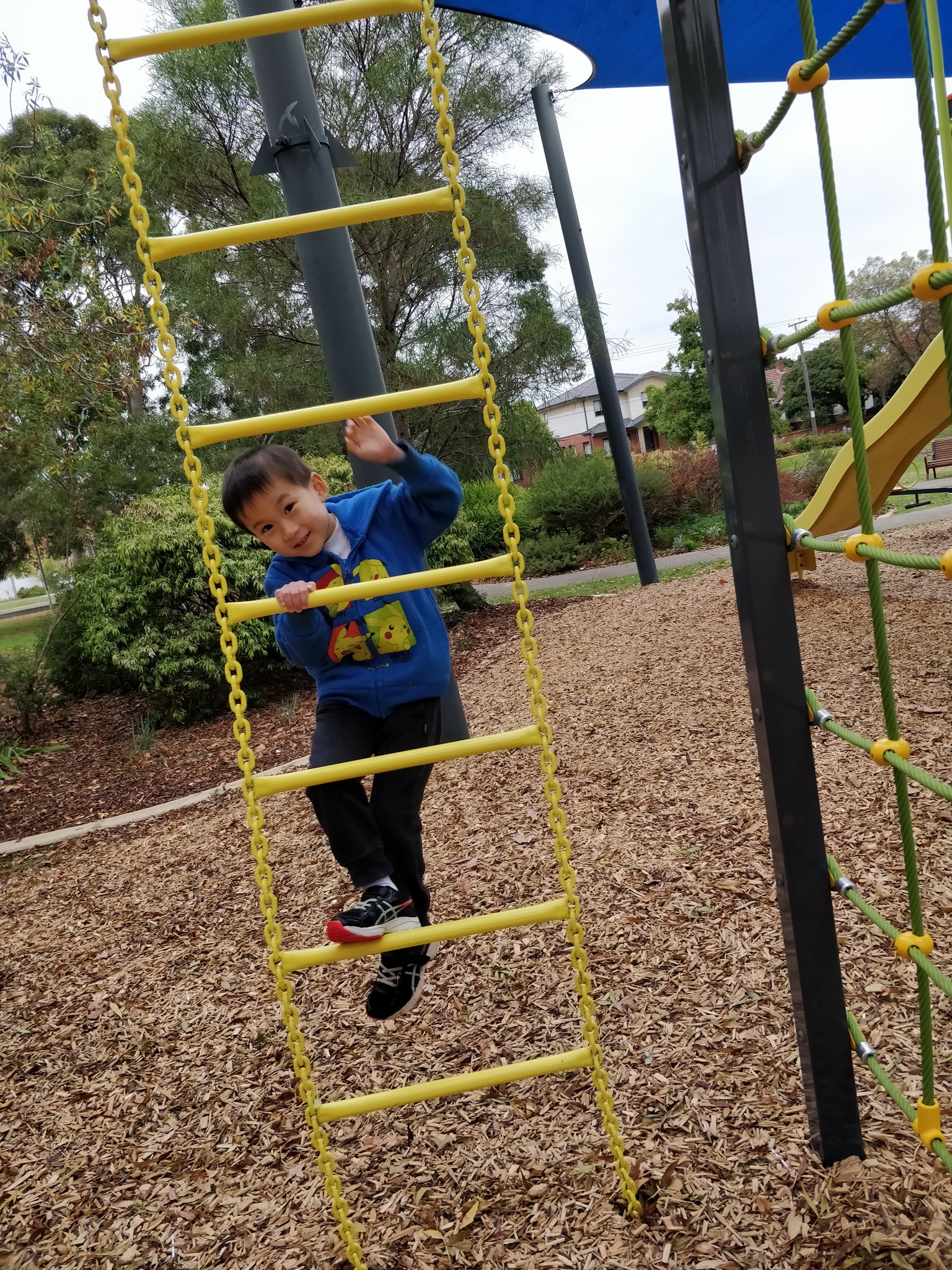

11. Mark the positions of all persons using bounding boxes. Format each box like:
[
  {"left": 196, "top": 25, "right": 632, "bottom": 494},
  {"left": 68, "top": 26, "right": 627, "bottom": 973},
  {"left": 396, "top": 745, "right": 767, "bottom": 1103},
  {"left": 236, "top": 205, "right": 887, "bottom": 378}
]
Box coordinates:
[{"left": 222, "top": 416, "right": 463, "bottom": 1020}]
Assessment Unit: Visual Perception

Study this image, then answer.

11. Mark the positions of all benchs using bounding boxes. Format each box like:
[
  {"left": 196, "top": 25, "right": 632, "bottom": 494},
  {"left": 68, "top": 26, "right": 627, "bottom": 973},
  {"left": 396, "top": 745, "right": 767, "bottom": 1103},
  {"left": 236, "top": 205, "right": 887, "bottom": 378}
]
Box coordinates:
[{"left": 924, "top": 437, "right": 952, "bottom": 481}]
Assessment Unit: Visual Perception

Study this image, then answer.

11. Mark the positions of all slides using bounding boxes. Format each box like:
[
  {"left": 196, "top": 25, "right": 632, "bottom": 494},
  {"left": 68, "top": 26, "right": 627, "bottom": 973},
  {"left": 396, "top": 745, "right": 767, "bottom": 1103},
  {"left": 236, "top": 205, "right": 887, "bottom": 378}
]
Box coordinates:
[{"left": 783, "top": 329, "right": 952, "bottom": 574}]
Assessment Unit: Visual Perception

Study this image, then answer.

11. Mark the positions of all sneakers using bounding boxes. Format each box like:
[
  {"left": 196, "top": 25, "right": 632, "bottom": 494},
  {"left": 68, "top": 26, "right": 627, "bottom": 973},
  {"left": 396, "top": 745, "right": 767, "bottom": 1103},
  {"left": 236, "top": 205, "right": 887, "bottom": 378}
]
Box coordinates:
[
  {"left": 327, "top": 883, "right": 420, "bottom": 940},
  {"left": 364, "top": 939, "right": 440, "bottom": 1020}
]
